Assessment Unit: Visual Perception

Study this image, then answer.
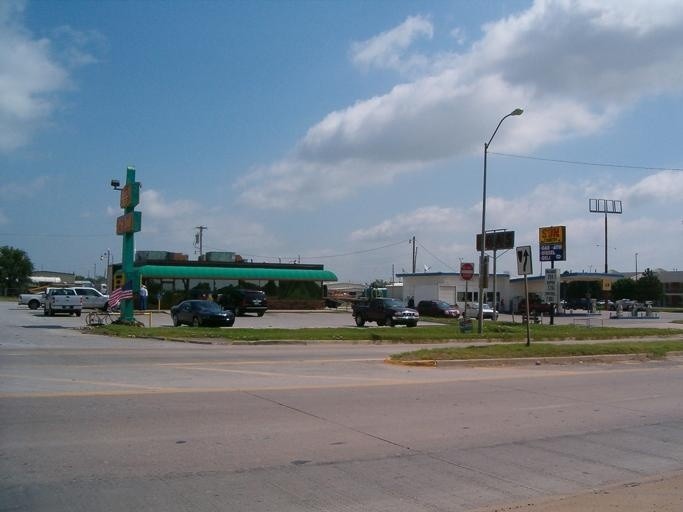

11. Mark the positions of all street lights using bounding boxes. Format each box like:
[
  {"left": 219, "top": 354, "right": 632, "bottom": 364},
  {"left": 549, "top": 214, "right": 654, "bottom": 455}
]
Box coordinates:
[
  {"left": 635, "top": 253, "right": 639, "bottom": 281},
  {"left": 478, "top": 107, "right": 524, "bottom": 332},
  {"left": 589, "top": 199, "right": 622, "bottom": 276}
]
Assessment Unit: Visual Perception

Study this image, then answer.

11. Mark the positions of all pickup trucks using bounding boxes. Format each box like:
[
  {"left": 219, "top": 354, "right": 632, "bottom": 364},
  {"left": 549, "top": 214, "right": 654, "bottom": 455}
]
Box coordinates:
[
  {"left": 19, "top": 287, "right": 119, "bottom": 318},
  {"left": 352, "top": 298, "right": 418, "bottom": 326}
]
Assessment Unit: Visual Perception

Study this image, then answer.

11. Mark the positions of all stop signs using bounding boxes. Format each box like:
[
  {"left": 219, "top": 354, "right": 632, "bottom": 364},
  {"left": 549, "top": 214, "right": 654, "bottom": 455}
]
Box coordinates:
[{"left": 462, "top": 263, "right": 475, "bottom": 280}]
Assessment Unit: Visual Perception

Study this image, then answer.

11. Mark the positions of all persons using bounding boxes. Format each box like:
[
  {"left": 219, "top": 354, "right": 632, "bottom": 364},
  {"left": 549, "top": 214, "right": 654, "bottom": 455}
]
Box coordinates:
[
  {"left": 202, "top": 293, "right": 214, "bottom": 301},
  {"left": 407, "top": 296, "right": 414, "bottom": 308},
  {"left": 501, "top": 299, "right": 505, "bottom": 311},
  {"left": 139, "top": 286, "right": 149, "bottom": 310}
]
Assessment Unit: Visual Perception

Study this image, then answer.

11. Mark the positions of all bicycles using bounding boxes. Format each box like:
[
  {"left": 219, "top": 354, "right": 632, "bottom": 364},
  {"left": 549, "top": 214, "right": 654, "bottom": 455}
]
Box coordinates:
[{"left": 85, "top": 308, "right": 119, "bottom": 325}]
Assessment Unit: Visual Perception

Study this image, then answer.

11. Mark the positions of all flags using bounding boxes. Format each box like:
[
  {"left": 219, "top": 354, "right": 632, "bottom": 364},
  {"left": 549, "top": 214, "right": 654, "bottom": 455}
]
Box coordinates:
[{"left": 108, "top": 280, "right": 133, "bottom": 308}]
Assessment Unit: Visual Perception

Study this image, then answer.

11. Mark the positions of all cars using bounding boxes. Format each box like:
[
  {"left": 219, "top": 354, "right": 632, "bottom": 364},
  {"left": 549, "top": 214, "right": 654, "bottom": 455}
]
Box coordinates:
[
  {"left": 418, "top": 301, "right": 499, "bottom": 320},
  {"left": 518, "top": 297, "right": 552, "bottom": 314},
  {"left": 168, "top": 289, "right": 267, "bottom": 327},
  {"left": 563, "top": 298, "right": 645, "bottom": 310}
]
